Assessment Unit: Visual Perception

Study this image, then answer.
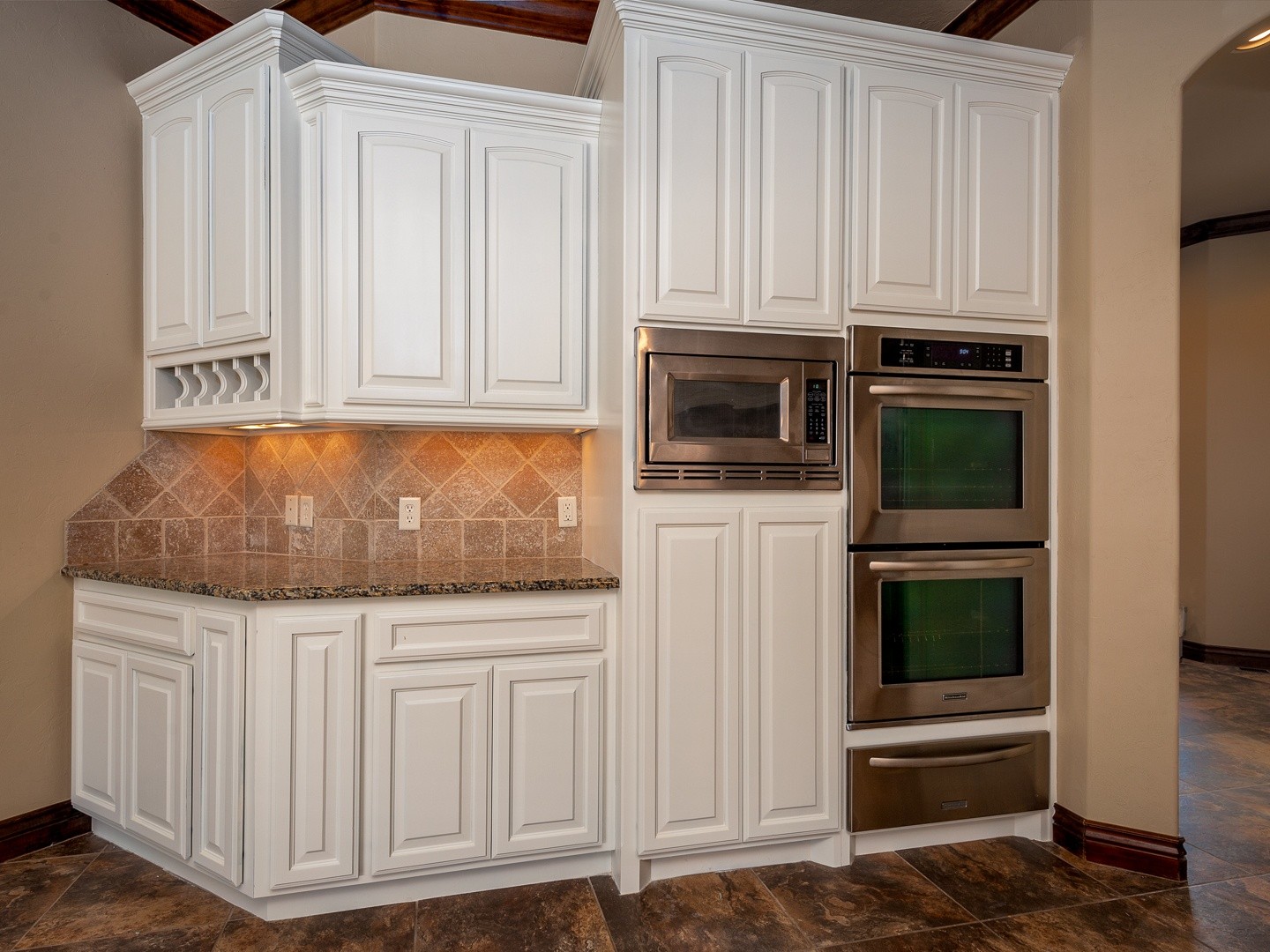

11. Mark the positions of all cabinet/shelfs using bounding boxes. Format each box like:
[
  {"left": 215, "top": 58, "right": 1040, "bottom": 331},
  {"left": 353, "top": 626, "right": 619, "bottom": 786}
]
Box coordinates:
[
  {"left": 364, "top": 600, "right": 620, "bottom": 877},
  {"left": 188, "top": 608, "right": 249, "bottom": 891},
  {"left": 258, "top": 608, "right": 367, "bottom": 889},
  {"left": 290, "top": 58, "right": 601, "bottom": 429},
  {"left": 849, "top": 18, "right": 1075, "bottom": 332},
  {"left": 128, "top": 11, "right": 289, "bottom": 432},
  {"left": 573, "top": 0, "right": 850, "bottom": 339},
  {"left": 72, "top": 589, "right": 196, "bottom": 859},
  {"left": 635, "top": 498, "right": 851, "bottom": 861}
]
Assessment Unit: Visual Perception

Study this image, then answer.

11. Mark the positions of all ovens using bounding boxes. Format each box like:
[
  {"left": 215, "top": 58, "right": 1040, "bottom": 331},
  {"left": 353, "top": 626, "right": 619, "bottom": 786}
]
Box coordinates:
[{"left": 848, "top": 323, "right": 1052, "bottom": 833}]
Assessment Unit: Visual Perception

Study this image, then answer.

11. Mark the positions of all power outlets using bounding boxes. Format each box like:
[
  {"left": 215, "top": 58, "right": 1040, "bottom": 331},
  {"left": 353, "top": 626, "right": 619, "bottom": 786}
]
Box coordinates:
[
  {"left": 284, "top": 496, "right": 298, "bottom": 526},
  {"left": 299, "top": 496, "right": 313, "bottom": 525},
  {"left": 398, "top": 496, "right": 421, "bottom": 530},
  {"left": 558, "top": 496, "right": 578, "bottom": 527}
]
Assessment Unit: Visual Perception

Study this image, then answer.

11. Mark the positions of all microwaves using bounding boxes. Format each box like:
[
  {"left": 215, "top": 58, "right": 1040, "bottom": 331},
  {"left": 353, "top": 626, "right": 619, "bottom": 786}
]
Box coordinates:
[{"left": 633, "top": 326, "right": 844, "bottom": 491}]
